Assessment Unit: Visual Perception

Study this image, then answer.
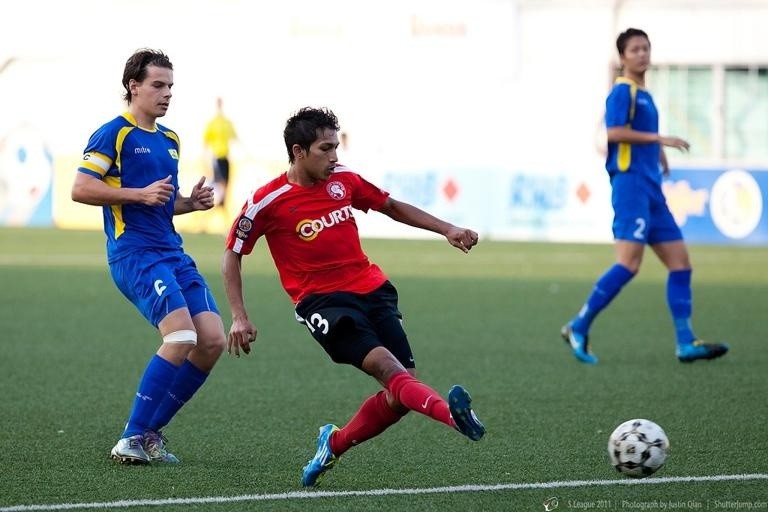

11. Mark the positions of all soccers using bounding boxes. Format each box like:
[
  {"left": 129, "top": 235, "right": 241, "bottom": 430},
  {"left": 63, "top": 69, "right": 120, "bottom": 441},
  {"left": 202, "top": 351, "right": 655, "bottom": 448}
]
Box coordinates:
[{"left": 607, "top": 416, "right": 671, "bottom": 479}]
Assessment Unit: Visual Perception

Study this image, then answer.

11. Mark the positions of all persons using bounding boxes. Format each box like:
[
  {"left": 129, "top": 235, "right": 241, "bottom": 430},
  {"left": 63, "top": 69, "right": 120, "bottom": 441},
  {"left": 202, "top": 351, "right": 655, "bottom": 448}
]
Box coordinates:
[
  {"left": 561, "top": 28, "right": 730, "bottom": 366},
  {"left": 202, "top": 97, "right": 238, "bottom": 212},
  {"left": 70, "top": 48, "right": 227, "bottom": 466},
  {"left": 221, "top": 106, "right": 487, "bottom": 488}
]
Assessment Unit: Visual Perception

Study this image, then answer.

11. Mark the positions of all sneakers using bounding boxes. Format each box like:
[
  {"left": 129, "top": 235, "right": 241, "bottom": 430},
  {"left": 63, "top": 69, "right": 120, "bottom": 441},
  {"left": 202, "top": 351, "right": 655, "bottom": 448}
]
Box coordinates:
[
  {"left": 301, "top": 423, "right": 341, "bottom": 487},
  {"left": 676, "top": 340, "right": 728, "bottom": 363},
  {"left": 110, "top": 430, "right": 181, "bottom": 464},
  {"left": 560, "top": 324, "right": 599, "bottom": 363},
  {"left": 448, "top": 385, "right": 485, "bottom": 441}
]
